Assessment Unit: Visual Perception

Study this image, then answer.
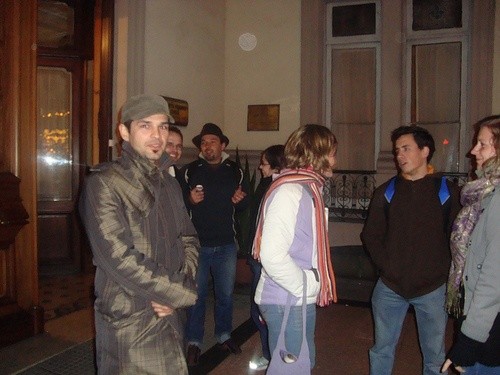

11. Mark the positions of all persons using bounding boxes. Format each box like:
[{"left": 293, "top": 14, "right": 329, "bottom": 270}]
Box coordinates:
[
  {"left": 165, "top": 126, "right": 192, "bottom": 219},
  {"left": 359, "top": 126, "right": 461, "bottom": 375},
  {"left": 252, "top": 124, "right": 338, "bottom": 371},
  {"left": 240, "top": 144, "right": 287, "bottom": 370},
  {"left": 77, "top": 94, "right": 202, "bottom": 375},
  {"left": 441, "top": 117, "right": 500, "bottom": 375},
  {"left": 177, "top": 123, "right": 250, "bottom": 368}
]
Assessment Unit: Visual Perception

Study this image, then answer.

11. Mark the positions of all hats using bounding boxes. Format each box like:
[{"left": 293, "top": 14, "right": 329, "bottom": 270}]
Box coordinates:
[
  {"left": 191, "top": 123, "right": 230, "bottom": 149},
  {"left": 120, "top": 95, "right": 175, "bottom": 123}
]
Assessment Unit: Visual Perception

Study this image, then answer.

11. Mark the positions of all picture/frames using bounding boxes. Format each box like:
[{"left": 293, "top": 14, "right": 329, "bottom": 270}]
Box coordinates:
[
  {"left": 161, "top": 96, "right": 189, "bottom": 127},
  {"left": 246, "top": 104, "right": 280, "bottom": 131}
]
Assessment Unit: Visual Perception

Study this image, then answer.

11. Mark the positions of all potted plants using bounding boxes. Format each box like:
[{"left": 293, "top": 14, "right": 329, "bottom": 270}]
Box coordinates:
[{"left": 234, "top": 144, "right": 259, "bottom": 255}]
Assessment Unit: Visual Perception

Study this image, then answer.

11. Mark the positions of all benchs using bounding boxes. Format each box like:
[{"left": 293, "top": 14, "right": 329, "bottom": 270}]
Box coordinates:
[{"left": 330, "top": 245, "right": 379, "bottom": 302}]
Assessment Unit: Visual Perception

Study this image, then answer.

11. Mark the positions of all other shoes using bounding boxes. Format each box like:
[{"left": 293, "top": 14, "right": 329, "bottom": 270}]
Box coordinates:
[
  {"left": 222, "top": 338, "right": 242, "bottom": 355},
  {"left": 185, "top": 344, "right": 202, "bottom": 365}
]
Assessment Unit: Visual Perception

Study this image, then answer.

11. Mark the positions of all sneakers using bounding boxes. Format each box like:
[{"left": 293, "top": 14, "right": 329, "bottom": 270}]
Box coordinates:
[{"left": 249, "top": 356, "right": 270, "bottom": 371}]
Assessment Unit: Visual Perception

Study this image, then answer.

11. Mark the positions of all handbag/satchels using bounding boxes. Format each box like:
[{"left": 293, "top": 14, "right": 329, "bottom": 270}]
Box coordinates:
[{"left": 266, "top": 333, "right": 310, "bottom": 375}]
